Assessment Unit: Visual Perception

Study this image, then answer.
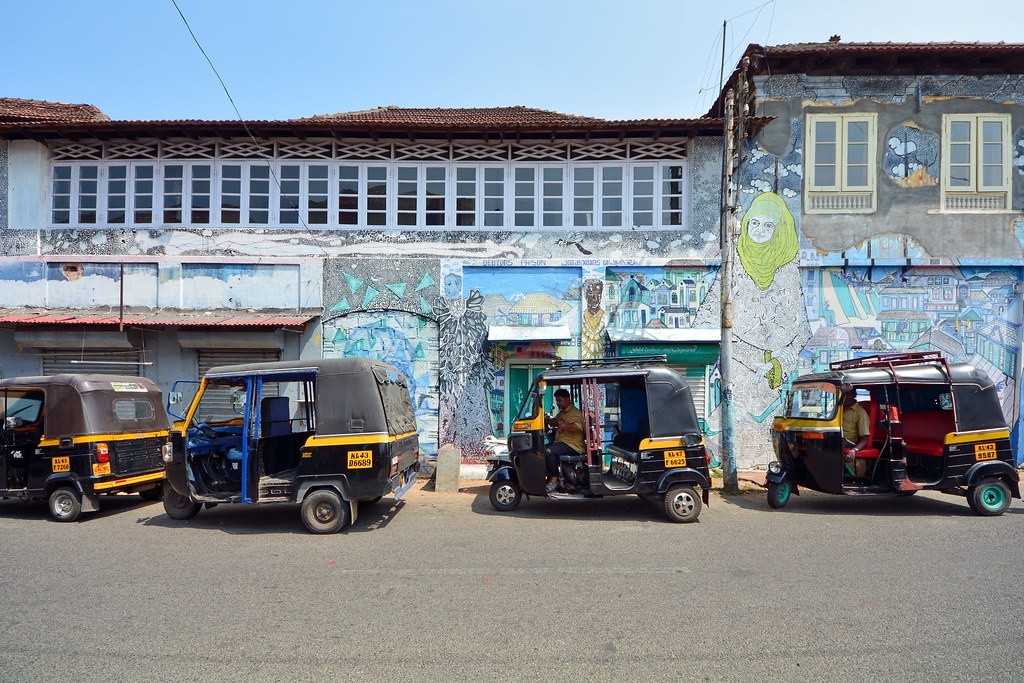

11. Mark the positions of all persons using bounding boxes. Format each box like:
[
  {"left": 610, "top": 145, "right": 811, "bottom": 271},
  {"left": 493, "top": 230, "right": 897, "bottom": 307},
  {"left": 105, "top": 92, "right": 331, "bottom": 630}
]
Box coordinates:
[
  {"left": 545, "top": 389, "right": 584, "bottom": 492},
  {"left": 842, "top": 388, "right": 870, "bottom": 465}
]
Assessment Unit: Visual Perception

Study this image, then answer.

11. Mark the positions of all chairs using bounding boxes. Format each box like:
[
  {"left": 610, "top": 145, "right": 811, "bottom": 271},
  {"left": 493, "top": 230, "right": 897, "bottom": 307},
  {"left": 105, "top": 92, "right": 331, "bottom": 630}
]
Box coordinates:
[
  {"left": 227, "top": 396, "right": 289, "bottom": 460},
  {"left": 558, "top": 414, "right": 599, "bottom": 462},
  {"left": 841, "top": 401, "right": 881, "bottom": 460}
]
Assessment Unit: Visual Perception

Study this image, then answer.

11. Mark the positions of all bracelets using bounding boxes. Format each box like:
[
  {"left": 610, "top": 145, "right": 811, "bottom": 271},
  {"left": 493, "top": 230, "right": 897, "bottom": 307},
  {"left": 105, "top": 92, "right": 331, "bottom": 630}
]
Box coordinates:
[{"left": 854, "top": 448, "right": 858, "bottom": 452}]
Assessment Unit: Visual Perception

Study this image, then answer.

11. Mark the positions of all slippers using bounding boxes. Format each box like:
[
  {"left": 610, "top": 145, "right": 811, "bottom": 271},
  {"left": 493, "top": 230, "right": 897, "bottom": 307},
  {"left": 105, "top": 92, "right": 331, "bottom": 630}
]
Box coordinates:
[{"left": 546, "top": 482, "right": 558, "bottom": 492}]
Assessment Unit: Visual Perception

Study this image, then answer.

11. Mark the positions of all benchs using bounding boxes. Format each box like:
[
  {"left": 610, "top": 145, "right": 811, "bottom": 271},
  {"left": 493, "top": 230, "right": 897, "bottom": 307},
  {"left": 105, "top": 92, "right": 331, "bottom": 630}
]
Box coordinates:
[
  {"left": 606, "top": 419, "right": 648, "bottom": 461},
  {"left": 877, "top": 411, "right": 956, "bottom": 456}
]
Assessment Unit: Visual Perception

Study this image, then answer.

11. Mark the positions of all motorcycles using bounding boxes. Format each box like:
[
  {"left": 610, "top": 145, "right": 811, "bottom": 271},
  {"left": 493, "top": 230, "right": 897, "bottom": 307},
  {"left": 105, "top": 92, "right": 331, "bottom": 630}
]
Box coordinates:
[
  {"left": 162, "top": 357, "right": 420, "bottom": 535},
  {"left": 485, "top": 355, "right": 712, "bottom": 523},
  {"left": 0, "top": 374, "right": 171, "bottom": 522},
  {"left": 766, "top": 351, "right": 1021, "bottom": 516}
]
